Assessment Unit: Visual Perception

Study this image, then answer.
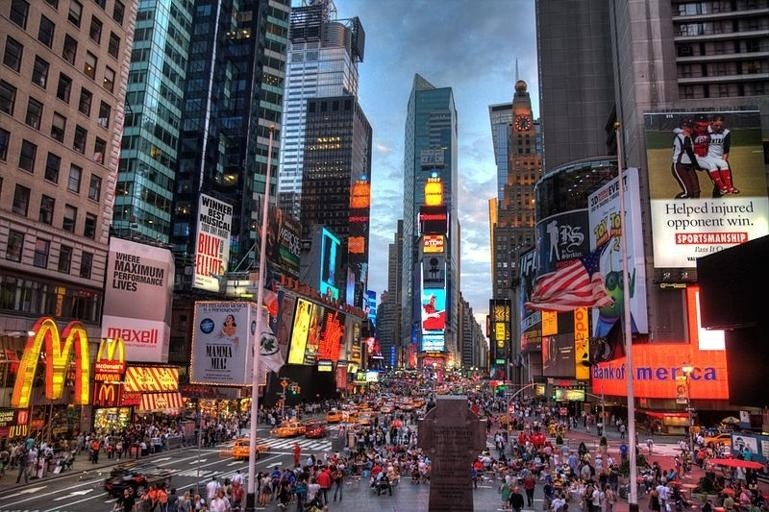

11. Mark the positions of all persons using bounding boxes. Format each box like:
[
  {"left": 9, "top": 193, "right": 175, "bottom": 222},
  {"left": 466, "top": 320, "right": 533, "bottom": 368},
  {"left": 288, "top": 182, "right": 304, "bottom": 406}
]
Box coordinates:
[
  {"left": 221, "top": 314, "right": 240, "bottom": 345},
  {"left": 707, "top": 115, "right": 732, "bottom": 198},
  {"left": 671, "top": 117, "right": 700, "bottom": 198},
  {"left": 0, "top": 396, "right": 769, "bottom": 512},
  {"left": 672, "top": 116, "right": 740, "bottom": 195}
]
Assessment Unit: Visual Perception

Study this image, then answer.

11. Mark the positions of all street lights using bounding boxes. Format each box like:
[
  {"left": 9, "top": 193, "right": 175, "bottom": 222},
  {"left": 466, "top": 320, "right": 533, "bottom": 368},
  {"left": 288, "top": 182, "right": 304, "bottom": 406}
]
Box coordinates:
[
  {"left": 552, "top": 387, "right": 607, "bottom": 439},
  {"left": 506, "top": 382, "right": 545, "bottom": 445},
  {"left": 681, "top": 365, "right": 696, "bottom": 465}
]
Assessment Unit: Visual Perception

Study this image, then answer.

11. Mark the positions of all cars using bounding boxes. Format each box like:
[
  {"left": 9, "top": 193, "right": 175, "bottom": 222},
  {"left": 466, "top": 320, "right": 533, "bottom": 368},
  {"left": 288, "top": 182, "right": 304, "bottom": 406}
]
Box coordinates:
[
  {"left": 271, "top": 419, "right": 329, "bottom": 439},
  {"left": 231, "top": 437, "right": 271, "bottom": 460},
  {"left": 325, "top": 399, "right": 426, "bottom": 427}
]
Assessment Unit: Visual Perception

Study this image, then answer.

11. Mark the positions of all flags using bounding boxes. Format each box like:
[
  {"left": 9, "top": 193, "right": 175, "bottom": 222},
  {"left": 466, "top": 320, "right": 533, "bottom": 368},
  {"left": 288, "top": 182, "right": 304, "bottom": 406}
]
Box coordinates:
[{"left": 524, "top": 238, "right": 618, "bottom": 314}]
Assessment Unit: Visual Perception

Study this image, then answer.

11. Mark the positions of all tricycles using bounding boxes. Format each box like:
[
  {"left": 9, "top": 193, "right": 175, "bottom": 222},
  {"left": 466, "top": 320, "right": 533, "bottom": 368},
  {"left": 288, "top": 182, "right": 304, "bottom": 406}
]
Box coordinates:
[{"left": 373, "top": 476, "right": 393, "bottom": 496}]
